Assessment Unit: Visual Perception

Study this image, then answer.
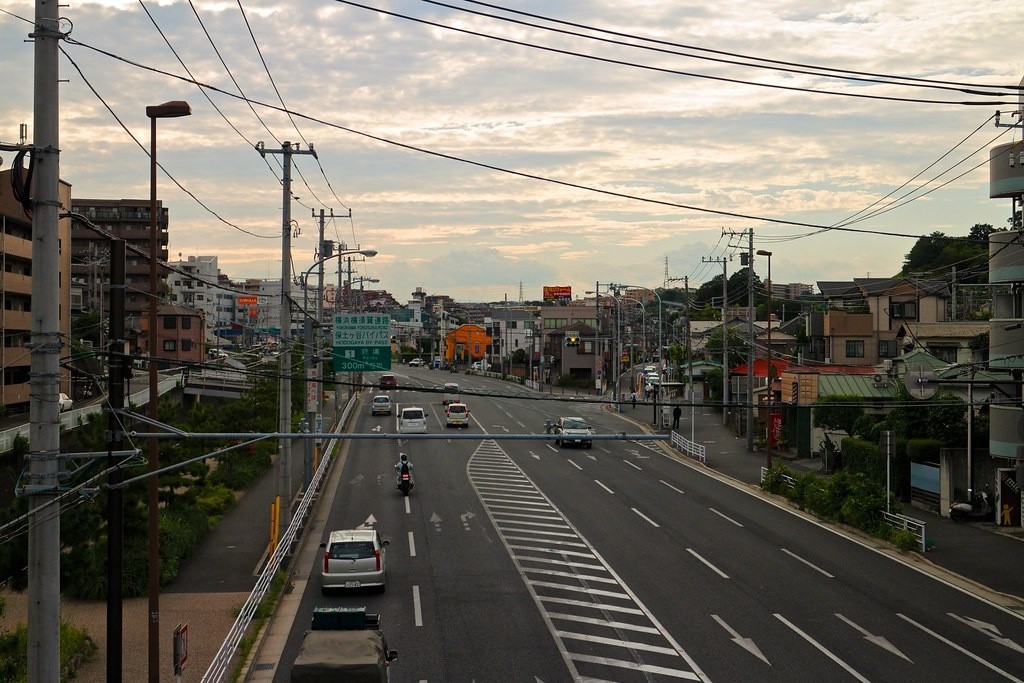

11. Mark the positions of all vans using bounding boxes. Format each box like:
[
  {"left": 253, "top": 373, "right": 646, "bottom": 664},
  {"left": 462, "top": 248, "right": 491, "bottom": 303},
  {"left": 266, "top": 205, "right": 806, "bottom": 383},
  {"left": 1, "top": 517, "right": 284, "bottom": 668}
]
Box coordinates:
[{"left": 442, "top": 383, "right": 460, "bottom": 404}]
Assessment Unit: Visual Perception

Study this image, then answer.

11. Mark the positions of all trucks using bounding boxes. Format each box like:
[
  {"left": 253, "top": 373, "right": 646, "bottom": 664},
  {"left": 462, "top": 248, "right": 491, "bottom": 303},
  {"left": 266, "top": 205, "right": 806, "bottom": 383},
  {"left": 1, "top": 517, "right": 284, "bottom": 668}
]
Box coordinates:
[
  {"left": 371, "top": 396, "right": 392, "bottom": 415},
  {"left": 289, "top": 606, "right": 398, "bottom": 683}
]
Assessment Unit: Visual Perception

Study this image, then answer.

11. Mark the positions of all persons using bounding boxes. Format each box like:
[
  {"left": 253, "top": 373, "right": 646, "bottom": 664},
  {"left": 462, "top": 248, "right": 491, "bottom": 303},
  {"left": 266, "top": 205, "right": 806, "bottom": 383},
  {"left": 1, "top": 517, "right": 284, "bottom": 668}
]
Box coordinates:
[
  {"left": 673, "top": 406, "right": 681, "bottom": 429},
  {"left": 395, "top": 455, "right": 414, "bottom": 485}
]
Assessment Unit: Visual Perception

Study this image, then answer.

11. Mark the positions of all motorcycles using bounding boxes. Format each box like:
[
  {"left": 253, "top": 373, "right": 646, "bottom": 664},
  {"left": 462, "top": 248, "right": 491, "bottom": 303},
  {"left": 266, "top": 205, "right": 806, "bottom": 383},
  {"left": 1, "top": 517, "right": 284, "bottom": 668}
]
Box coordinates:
[{"left": 394, "top": 465, "right": 414, "bottom": 496}]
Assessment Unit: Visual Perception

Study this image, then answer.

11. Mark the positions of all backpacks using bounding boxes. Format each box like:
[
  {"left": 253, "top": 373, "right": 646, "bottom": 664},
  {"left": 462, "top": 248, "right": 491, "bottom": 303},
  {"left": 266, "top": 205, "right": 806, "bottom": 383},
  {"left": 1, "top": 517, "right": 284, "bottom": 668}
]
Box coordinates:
[{"left": 401, "top": 462, "right": 409, "bottom": 475}]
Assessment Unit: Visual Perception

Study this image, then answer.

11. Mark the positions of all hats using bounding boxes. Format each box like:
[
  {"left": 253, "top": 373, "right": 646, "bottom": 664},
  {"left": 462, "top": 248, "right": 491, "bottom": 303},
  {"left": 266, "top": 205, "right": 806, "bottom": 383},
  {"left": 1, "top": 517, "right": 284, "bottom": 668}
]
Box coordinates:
[{"left": 401, "top": 455, "right": 407, "bottom": 461}]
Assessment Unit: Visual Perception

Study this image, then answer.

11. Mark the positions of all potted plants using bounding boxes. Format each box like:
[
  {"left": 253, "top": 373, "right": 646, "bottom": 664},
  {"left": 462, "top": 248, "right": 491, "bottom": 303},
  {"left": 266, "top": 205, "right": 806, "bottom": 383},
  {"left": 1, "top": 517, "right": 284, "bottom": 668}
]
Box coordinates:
[{"left": 777, "top": 424, "right": 794, "bottom": 452}]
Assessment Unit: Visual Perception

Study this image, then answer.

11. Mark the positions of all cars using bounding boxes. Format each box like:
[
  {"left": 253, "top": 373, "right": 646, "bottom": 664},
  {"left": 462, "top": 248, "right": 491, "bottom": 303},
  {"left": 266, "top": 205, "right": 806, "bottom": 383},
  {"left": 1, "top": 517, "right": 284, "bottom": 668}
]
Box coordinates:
[
  {"left": 445, "top": 403, "right": 470, "bottom": 428},
  {"left": 378, "top": 375, "right": 397, "bottom": 390},
  {"left": 397, "top": 408, "right": 428, "bottom": 433},
  {"left": 409, "top": 358, "right": 492, "bottom": 371},
  {"left": 59, "top": 392, "right": 73, "bottom": 413},
  {"left": 645, "top": 355, "right": 660, "bottom": 382},
  {"left": 207, "top": 349, "right": 228, "bottom": 359},
  {"left": 555, "top": 416, "right": 592, "bottom": 448},
  {"left": 319, "top": 529, "right": 390, "bottom": 595}
]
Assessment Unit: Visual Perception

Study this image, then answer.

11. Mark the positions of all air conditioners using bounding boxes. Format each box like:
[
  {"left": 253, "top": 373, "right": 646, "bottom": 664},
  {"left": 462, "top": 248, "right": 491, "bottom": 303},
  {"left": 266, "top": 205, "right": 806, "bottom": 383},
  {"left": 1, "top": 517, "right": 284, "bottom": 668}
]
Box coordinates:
[{"left": 882, "top": 360, "right": 893, "bottom": 370}]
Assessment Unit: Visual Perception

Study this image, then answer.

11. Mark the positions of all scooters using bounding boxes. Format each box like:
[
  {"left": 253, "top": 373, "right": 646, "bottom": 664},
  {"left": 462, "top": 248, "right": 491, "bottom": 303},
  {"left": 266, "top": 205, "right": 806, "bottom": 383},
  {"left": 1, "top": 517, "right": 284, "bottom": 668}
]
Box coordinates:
[{"left": 947, "top": 483, "right": 994, "bottom": 521}]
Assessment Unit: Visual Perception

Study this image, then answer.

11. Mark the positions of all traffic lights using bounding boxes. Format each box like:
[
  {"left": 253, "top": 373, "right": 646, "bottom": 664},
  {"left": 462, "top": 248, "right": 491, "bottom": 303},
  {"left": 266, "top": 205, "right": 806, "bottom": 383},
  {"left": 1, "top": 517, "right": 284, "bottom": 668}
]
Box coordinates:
[{"left": 566, "top": 337, "right": 581, "bottom": 347}]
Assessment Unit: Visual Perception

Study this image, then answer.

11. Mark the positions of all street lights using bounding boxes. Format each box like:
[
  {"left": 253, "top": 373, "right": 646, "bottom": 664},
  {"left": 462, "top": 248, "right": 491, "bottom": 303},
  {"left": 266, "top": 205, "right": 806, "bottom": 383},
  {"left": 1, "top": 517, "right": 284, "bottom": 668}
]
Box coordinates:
[
  {"left": 304, "top": 250, "right": 381, "bottom": 493},
  {"left": 615, "top": 297, "right": 647, "bottom": 374},
  {"left": 585, "top": 291, "right": 622, "bottom": 412},
  {"left": 146, "top": 101, "right": 191, "bottom": 683},
  {"left": 652, "top": 318, "right": 675, "bottom": 347},
  {"left": 617, "top": 284, "right": 662, "bottom": 430},
  {"left": 756, "top": 250, "right": 772, "bottom": 469}
]
